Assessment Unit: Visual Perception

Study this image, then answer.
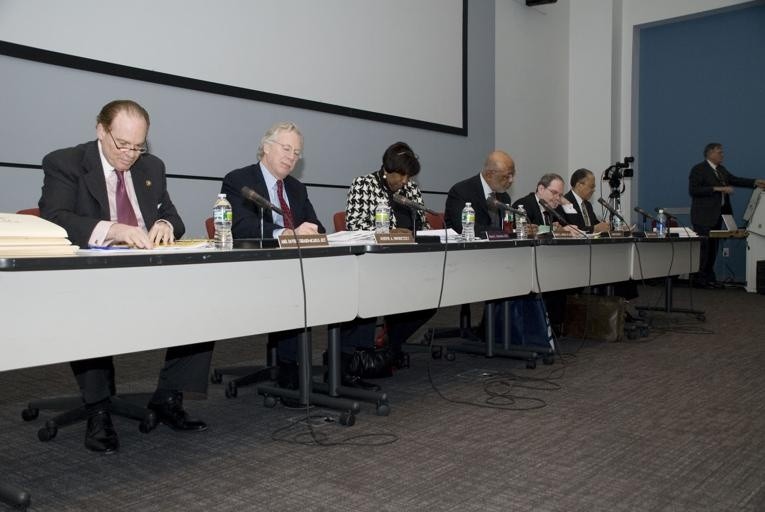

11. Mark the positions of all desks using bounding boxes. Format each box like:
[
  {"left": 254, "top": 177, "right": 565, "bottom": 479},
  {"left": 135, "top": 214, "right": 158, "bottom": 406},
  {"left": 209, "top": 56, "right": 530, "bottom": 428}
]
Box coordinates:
[
  {"left": 0, "top": 238, "right": 363, "bottom": 512},
  {"left": 445, "top": 236, "right": 633, "bottom": 365},
  {"left": 611, "top": 235, "right": 706, "bottom": 337},
  {"left": 287, "top": 236, "right": 539, "bottom": 417}
]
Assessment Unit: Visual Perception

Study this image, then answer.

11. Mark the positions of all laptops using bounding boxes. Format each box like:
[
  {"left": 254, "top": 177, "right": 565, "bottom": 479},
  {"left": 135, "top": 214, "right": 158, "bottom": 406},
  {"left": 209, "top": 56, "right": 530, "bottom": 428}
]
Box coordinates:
[{"left": 710, "top": 214, "right": 738, "bottom": 232}]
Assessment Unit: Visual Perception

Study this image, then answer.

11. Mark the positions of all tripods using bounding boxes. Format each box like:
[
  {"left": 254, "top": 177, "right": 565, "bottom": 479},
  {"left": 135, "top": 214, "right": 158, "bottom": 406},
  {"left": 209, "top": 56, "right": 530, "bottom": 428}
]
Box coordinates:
[{"left": 600, "top": 180, "right": 624, "bottom": 227}]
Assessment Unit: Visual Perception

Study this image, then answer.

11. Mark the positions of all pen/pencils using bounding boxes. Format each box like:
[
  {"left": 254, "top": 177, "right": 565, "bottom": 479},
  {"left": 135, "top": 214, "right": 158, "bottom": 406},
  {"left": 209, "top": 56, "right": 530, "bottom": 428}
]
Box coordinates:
[{"left": 90, "top": 245, "right": 130, "bottom": 249}]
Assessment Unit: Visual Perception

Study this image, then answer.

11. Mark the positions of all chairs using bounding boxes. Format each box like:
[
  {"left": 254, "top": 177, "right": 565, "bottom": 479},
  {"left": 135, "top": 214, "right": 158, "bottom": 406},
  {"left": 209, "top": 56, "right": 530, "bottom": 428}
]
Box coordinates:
[
  {"left": 332, "top": 210, "right": 444, "bottom": 370},
  {"left": 202, "top": 216, "right": 332, "bottom": 406},
  {"left": 16, "top": 208, "right": 159, "bottom": 454},
  {"left": 424, "top": 211, "right": 506, "bottom": 344}
]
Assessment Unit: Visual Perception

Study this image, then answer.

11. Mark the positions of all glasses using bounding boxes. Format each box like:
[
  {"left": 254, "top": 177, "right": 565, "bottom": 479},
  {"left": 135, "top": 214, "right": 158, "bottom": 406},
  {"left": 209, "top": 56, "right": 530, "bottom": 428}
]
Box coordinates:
[
  {"left": 547, "top": 188, "right": 564, "bottom": 198},
  {"left": 269, "top": 140, "right": 303, "bottom": 158},
  {"left": 108, "top": 128, "right": 149, "bottom": 154}
]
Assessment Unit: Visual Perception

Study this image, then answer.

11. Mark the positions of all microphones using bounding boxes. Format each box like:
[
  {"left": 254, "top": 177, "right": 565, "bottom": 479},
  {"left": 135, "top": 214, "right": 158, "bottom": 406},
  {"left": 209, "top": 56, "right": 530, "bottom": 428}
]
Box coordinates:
[
  {"left": 241, "top": 186, "right": 285, "bottom": 218},
  {"left": 655, "top": 208, "right": 677, "bottom": 219},
  {"left": 393, "top": 194, "right": 441, "bottom": 217},
  {"left": 598, "top": 198, "right": 623, "bottom": 221},
  {"left": 635, "top": 207, "right": 659, "bottom": 223},
  {"left": 487, "top": 197, "right": 525, "bottom": 217},
  {"left": 539, "top": 199, "right": 567, "bottom": 224}
]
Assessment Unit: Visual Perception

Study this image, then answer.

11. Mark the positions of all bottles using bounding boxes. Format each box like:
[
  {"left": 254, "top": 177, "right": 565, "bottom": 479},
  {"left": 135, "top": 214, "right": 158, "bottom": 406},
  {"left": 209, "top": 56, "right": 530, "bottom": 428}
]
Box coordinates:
[
  {"left": 612, "top": 209, "right": 624, "bottom": 238},
  {"left": 514, "top": 204, "right": 530, "bottom": 240},
  {"left": 654, "top": 209, "right": 669, "bottom": 238},
  {"left": 374, "top": 196, "right": 391, "bottom": 242},
  {"left": 460, "top": 202, "right": 476, "bottom": 242},
  {"left": 213, "top": 193, "right": 234, "bottom": 251},
  {"left": 501, "top": 203, "right": 515, "bottom": 240}
]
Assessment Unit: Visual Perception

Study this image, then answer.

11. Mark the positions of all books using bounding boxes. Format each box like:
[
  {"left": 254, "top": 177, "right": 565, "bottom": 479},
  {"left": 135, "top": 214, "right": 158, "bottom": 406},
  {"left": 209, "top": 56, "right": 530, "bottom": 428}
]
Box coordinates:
[{"left": 326, "top": 230, "right": 377, "bottom": 243}]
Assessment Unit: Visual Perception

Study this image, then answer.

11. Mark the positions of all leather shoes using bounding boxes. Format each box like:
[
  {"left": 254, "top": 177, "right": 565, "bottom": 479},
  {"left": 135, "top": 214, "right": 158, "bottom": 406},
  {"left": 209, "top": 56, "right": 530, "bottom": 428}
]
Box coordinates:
[
  {"left": 623, "top": 311, "right": 643, "bottom": 323},
  {"left": 323, "top": 370, "right": 382, "bottom": 393},
  {"left": 147, "top": 391, "right": 207, "bottom": 433},
  {"left": 84, "top": 410, "right": 119, "bottom": 457},
  {"left": 384, "top": 329, "right": 408, "bottom": 370},
  {"left": 277, "top": 379, "right": 312, "bottom": 408}
]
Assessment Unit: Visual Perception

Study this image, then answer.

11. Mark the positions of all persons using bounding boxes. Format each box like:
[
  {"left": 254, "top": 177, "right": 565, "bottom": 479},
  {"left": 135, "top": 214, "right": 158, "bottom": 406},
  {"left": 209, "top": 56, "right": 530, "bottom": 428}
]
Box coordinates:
[
  {"left": 511, "top": 172, "right": 582, "bottom": 231},
  {"left": 344, "top": 141, "right": 433, "bottom": 344},
  {"left": 687, "top": 142, "right": 764, "bottom": 288},
  {"left": 443, "top": 150, "right": 538, "bottom": 238},
  {"left": 219, "top": 121, "right": 381, "bottom": 408},
  {"left": 38, "top": 99, "right": 208, "bottom": 454},
  {"left": 551, "top": 168, "right": 639, "bottom": 301}
]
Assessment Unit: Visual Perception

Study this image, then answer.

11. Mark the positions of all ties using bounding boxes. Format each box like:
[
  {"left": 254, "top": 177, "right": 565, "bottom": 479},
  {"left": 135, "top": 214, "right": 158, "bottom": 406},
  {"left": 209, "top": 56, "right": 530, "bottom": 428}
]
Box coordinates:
[
  {"left": 115, "top": 168, "right": 138, "bottom": 227},
  {"left": 580, "top": 200, "right": 590, "bottom": 226},
  {"left": 543, "top": 211, "right": 551, "bottom": 226},
  {"left": 277, "top": 179, "right": 296, "bottom": 229}
]
